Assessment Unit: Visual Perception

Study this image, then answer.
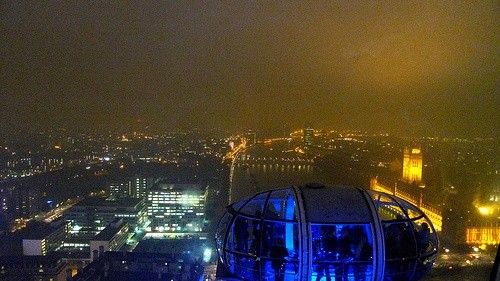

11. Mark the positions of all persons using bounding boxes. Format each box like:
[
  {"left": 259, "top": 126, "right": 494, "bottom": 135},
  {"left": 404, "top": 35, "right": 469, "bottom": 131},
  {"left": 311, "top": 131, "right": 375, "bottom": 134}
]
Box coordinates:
[{"left": 230, "top": 209, "right": 431, "bottom": 281}]
